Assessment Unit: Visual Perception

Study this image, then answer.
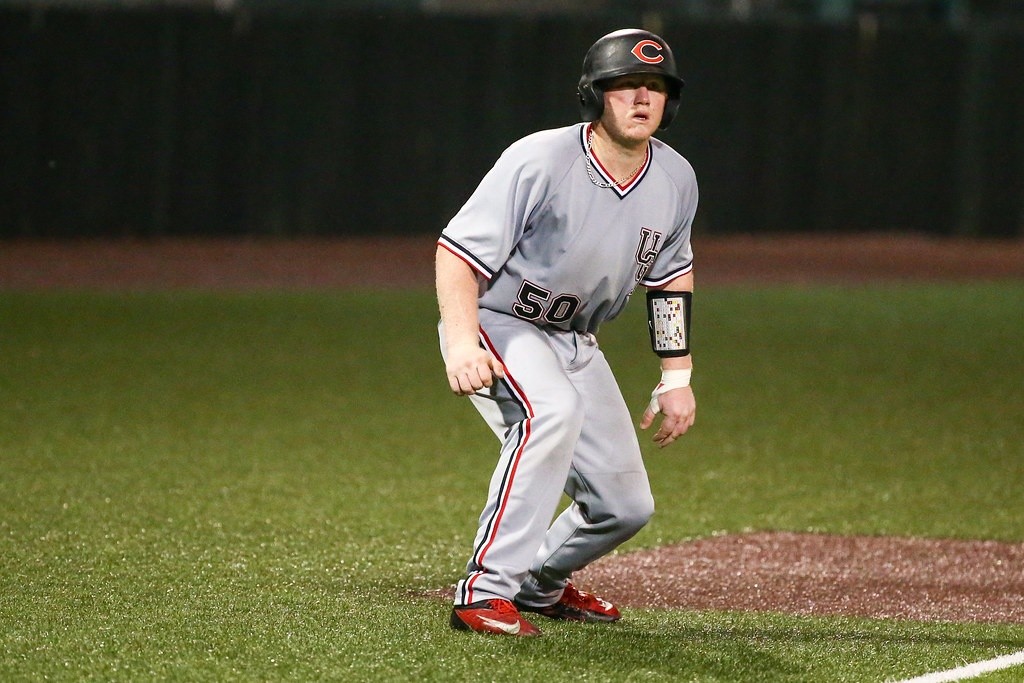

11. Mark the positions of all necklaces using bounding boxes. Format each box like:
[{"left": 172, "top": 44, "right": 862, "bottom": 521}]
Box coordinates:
[{"left": 586, "top": 119, "right": 647, "bottom": 188}]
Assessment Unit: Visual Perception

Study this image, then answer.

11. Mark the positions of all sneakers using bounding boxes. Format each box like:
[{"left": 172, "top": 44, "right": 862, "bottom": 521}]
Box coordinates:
[
  {"left": 448, "top": 599, "right": 544, "bottom": 640},
  {"left": 514, "top": 580, "right": 623, "bottom": 625}
]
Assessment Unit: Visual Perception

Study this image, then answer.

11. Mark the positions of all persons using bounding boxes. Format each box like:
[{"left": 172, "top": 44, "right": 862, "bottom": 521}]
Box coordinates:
[{"left": 435, "top": 29, "right": 700, "bottom": 636}]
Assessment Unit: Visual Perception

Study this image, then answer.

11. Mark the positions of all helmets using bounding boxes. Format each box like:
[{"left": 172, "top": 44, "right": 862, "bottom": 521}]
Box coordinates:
[{"left": 575, "top": 28, "right": 685, "bottom": 132}]
